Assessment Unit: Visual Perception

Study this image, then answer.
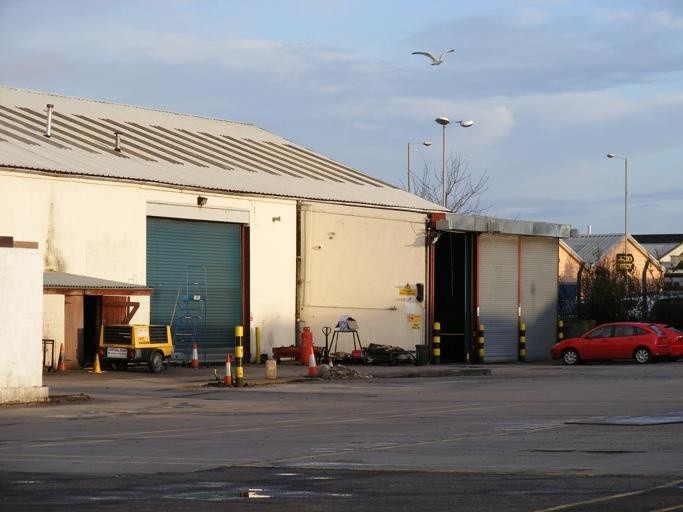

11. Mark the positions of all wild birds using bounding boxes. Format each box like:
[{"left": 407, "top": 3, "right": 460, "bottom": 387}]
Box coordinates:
[{"left": 411, "top": 48, "right": 454, "bottom": 65}]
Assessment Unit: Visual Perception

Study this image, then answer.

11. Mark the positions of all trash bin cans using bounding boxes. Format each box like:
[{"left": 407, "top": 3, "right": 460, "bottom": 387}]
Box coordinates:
[{"left": 415, "top": 345, "right": 425, "bottom": 366}]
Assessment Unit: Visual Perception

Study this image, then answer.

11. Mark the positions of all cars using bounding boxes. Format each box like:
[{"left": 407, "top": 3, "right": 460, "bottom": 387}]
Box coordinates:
[
  {"left": 614, "top": 297, "right": 656, "bottom": 321},
  {"left": 548, "top": 321, "right": 668, "bottom": 366},
  {"left": 647, "top": 324, "right": 682, "bottom": 361}
]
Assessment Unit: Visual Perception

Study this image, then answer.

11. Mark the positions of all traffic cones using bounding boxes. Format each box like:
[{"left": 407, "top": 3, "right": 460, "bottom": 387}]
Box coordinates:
[
  {"left": 327, "top": 354, "right": 333, "bottom": 367},
  {"left": 190, "top": 343, "right": 200, "bottom": 367},
  {"left": 303, "top": 345, "right": 321, "bottom": 378},
  {"left": 56, "top": 343, "right": 66, "bottom": 372},
  {"left": 90, "top": 352, "right": 104, "bottom": 374},
  {"left": 222, "top": 352, "right": 234, "bottom": 386}
]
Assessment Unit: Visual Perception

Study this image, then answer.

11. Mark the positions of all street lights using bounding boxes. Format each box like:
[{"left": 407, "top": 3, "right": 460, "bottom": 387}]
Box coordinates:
[
  {"left": 406, "top": 140, "right": 432, "bottom": 194},
  {"left": 605, "top": 152, "right": 629, "bottom": 292},
  {"left": 435, "top": 118, "right": 475, "bottom": 207}
]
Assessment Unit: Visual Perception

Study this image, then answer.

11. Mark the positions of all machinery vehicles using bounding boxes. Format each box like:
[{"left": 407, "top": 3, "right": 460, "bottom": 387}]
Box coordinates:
[{"left": 95, "top": 322, "right": 175, "bottom": 374}]
